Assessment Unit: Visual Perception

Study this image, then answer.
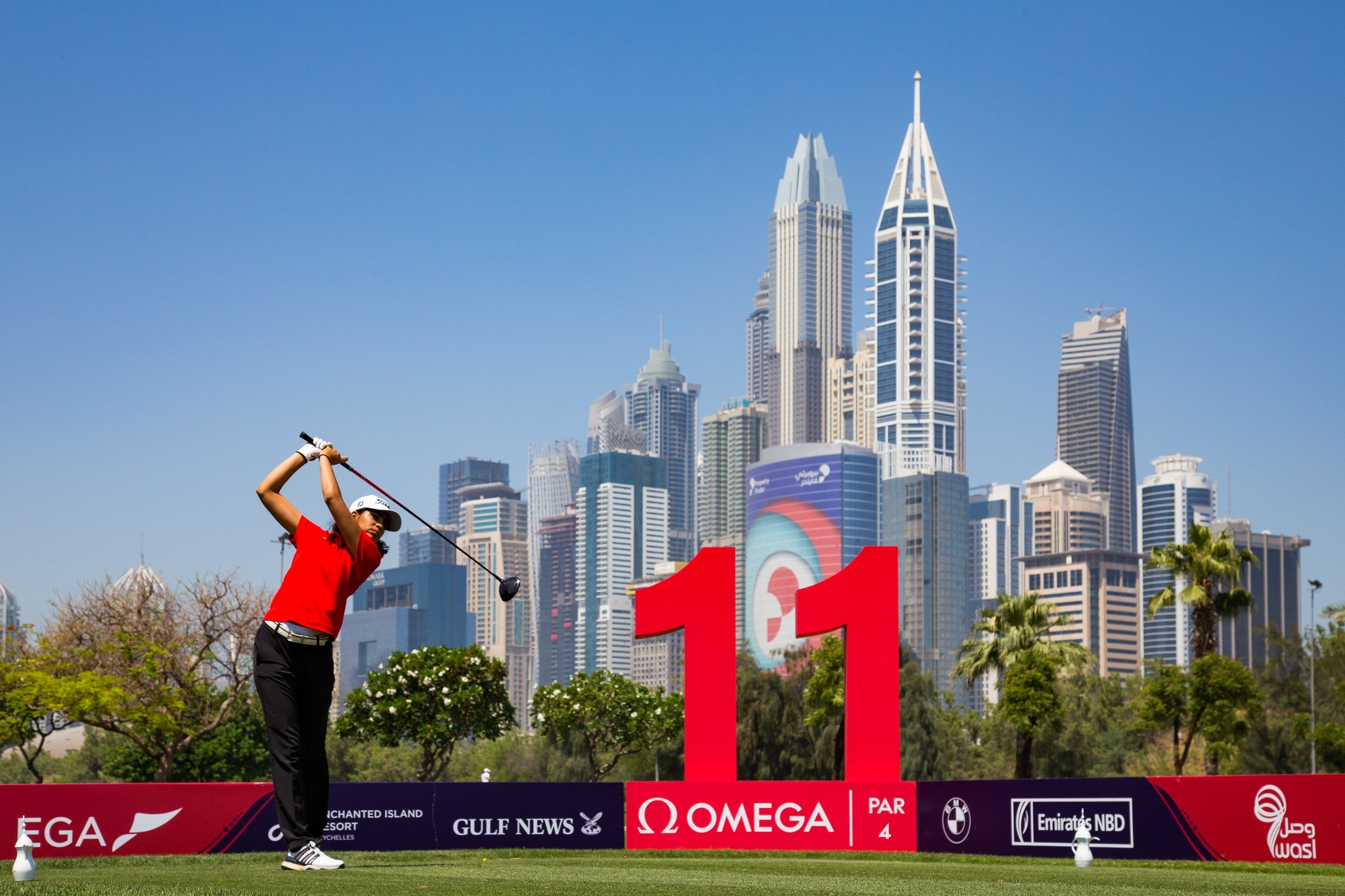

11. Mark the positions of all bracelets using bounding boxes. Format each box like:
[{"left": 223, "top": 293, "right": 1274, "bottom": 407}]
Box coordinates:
[{"left": 319, "top": 454, "right": 331, "bottom": 463}]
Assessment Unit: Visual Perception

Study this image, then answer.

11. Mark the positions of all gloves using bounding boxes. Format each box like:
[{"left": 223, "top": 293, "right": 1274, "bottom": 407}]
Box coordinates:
[{"left": 297, "top": 438, "right": 334, "bottom": 464}]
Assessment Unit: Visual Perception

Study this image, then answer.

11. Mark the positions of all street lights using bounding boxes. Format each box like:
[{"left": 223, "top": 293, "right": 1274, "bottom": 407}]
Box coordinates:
[{"left": 1307, "top": 578, "right": 1322, "bottom": 774}]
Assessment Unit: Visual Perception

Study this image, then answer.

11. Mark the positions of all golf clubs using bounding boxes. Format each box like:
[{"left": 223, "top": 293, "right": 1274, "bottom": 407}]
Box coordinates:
[{"left": 299, "top": 431, "right": 522, "bottom": 604}]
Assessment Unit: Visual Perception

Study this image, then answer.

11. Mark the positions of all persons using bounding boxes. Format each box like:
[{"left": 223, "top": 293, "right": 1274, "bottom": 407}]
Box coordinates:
[
  {"left": 481, "top": 768, "right": 491, "bottom": 782},
  {"left": 253, "top": 437, "right": 402, "bottom": 871}
]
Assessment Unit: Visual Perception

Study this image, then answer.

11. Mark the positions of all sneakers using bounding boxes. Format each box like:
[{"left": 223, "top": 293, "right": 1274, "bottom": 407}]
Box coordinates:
[{"left": 281, "top": 840, "right": 344, "bottom": 870}]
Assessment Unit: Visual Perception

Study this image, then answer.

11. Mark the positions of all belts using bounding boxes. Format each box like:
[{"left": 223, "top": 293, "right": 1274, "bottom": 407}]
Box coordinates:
[{"left": 264, "top": 620, "right": 329, "bottom": 646}]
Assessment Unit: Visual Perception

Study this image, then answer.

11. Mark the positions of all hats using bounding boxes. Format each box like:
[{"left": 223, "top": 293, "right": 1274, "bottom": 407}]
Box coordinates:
[
  {"left": 348, "top": 495, "right": 401, "bottom": 532},
  {"left": 483, "top": 768, "right": 491, "bottom": 772}
]
